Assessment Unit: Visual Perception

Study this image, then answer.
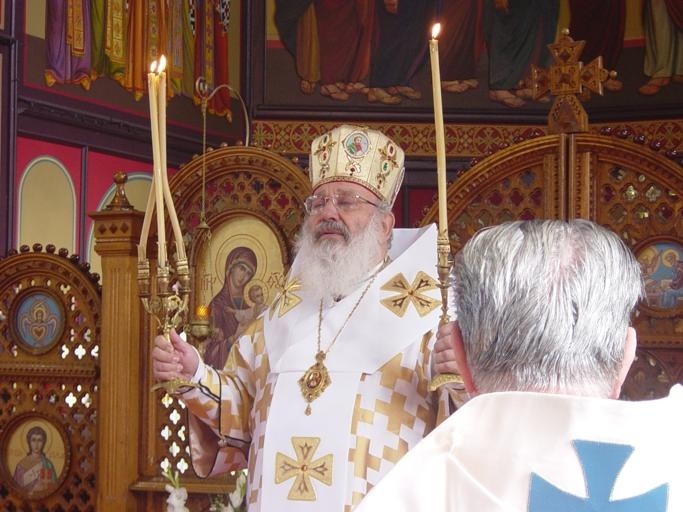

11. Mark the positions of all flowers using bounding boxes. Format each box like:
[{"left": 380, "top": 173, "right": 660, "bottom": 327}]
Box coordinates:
[{"left": 157, "top": 454, "right": 249, "bottom": 512}]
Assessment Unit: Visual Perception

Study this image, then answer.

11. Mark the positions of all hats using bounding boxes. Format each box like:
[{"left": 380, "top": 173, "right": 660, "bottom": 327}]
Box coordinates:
[{"left": 309, "top": 123, "right": 405, "bottom": 209}]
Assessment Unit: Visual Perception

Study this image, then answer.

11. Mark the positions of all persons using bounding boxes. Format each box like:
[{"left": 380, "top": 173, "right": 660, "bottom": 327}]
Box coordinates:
[
  {"left": 635, "top": 241, "right": 682, "bottom": 307},
  {"left": 12, "top": 424, "right": 58, "bottom": 494},
  {"left": 41, "top": 0, "right": 234, "bottom": 123},
  {"left": 202, "top": 242, "right": 266, "bottom": 369},
  {"left": 348, "top": 217, "right": 681, "bottom": 511},
  {"left": 149, "top": 126, "right": 474, "bottom": 511}
]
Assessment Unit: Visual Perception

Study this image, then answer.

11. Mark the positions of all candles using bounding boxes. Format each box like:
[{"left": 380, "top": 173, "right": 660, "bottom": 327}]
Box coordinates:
[
  {"left": 131, "top": 51, "right": 190, "bottom": 274},
  {"left": 418, "top": 20, "right": 454, "bottom": 248}
]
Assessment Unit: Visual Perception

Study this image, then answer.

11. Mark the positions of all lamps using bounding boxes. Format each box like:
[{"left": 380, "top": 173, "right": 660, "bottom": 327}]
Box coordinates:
[{"left": 186, "top": 75, "right": 250, "bottom": 343}]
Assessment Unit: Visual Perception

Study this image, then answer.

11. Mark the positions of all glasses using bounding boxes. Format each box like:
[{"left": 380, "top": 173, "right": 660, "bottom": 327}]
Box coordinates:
[{"left": 303, "top": 193, "right": 380, "bottom": 216}]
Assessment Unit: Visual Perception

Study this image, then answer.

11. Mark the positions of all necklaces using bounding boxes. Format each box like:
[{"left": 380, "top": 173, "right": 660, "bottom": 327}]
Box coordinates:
[{"left": 295, "top": 257, "right": 391, "bottom": 418}]
